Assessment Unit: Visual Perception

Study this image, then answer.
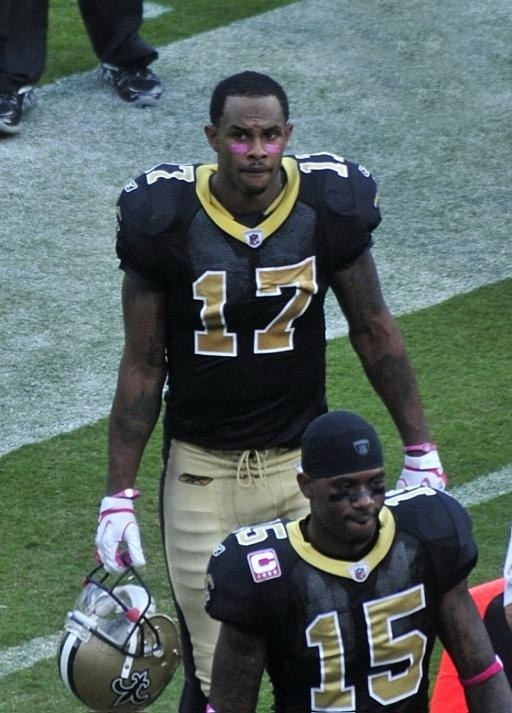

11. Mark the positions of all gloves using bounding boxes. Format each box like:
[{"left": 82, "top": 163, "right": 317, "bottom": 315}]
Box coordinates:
[
  {"left": 394, "top": 450, "right": 447, "bottom": 493},
  {"left": 94, "top": 496, "right": 146, "bottom": 574}
]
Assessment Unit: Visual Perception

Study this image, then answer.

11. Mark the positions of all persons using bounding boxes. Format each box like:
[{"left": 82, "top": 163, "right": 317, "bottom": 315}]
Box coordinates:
[
  {"left": 502, "top": 526, "right": 512, "bottom": 629},
  {"left": 0, "top": 0, "right": 162, "bottom": 135},
  {"left": 95, "top": 69, "right": 448, "bottom": 713},
  {"left": 203, "top": 409, "right": 512, "bottom": 713}
]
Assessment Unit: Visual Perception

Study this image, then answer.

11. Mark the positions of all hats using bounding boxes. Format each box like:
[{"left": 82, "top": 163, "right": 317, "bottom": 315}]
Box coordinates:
[{"left": 300, "top": 410, "right": 384, "bottom": 479}]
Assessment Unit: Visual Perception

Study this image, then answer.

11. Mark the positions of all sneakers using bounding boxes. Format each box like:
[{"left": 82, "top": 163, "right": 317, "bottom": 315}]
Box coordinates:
[
  {"left": 95, "top": 59, "right": 162, "bottom": 107},
  {"left": 0, "top": 84, "right": 39, "bottom": 137}
]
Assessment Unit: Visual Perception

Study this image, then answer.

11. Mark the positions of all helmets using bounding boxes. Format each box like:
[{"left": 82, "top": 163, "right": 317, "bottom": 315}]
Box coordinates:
[{"left": 56, "top": 556, "right": 183, "bottom": 713}]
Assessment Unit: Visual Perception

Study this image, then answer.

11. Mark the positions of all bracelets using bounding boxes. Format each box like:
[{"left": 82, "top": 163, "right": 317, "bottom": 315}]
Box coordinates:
[
  {"left": 112, "top": 488, "right": 141, "bottom": 498},
  {"left": 402, "top": 442, "right": 438, "bottom": 453}
]
